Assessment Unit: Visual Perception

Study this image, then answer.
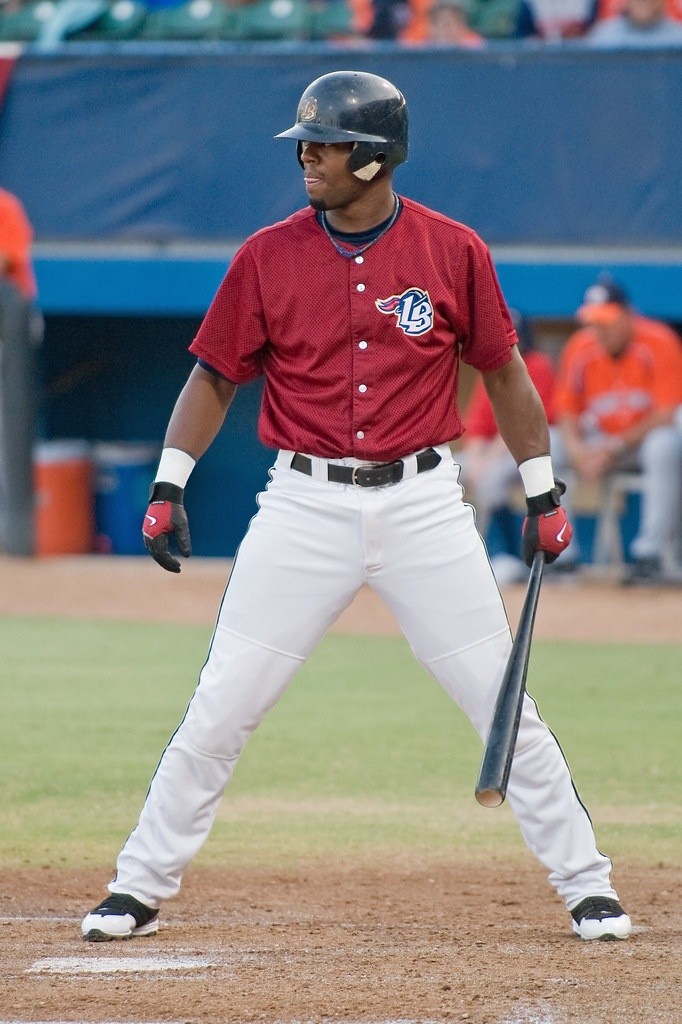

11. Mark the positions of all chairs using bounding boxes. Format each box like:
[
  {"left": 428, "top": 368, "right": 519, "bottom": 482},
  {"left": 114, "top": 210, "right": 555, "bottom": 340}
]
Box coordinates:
[{"left": 508, "top": 471, "right": 643, "bottom": 576}]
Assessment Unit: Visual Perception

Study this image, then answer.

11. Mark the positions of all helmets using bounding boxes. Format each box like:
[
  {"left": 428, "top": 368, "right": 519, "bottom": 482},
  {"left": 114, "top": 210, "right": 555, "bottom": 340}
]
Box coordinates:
[{"left": 273, "top": 71, "right": 409, "bottom": 184}]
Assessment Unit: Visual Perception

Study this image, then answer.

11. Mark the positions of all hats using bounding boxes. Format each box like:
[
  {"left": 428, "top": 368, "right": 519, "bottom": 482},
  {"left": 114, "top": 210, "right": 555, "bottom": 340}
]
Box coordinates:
[{"left": 575, "top": 284, "right": 638, "bottom": 322}]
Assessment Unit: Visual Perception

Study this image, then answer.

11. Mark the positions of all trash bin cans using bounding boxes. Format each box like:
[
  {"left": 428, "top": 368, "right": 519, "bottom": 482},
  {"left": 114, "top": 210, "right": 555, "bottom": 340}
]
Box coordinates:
[
  {"left": 95, "top": 443, "right": 164, "bottom": 553},
  {"left": 31, "top": 439, "right": 92, "bottom": 554}
]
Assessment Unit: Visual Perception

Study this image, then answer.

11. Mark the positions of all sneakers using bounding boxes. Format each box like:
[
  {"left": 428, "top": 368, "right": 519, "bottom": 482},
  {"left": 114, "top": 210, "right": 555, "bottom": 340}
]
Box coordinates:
[
  {"left": 82, "top": 891, "right": 159, "bottom": 942},
  {"left": 570, "top": 894, "right": 633, "bottom": 942}
]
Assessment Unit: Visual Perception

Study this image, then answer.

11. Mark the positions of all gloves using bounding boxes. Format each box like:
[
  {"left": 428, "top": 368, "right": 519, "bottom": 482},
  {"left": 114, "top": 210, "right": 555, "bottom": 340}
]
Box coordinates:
[
  {"left": 517, "top": 452, "right": 573, "bottom": 568},
  {"left": 142, "top": 448, "right": 197, "bottom": 573}
]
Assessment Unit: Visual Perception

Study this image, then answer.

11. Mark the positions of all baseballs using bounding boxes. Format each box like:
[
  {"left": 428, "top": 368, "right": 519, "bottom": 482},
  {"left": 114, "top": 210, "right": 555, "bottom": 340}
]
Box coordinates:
[{"left": 473, "top": 479, "right": 572, "bottom": 807}]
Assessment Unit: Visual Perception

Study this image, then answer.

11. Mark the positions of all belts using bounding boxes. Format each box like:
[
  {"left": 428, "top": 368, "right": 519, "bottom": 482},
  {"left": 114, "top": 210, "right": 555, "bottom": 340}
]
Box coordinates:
[{"left": 291, "top": 447, "right": 442, "bottom": 487}]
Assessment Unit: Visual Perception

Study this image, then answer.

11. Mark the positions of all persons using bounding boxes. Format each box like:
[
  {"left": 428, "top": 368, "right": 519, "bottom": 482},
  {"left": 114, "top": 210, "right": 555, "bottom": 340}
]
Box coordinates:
[
  {"left": 0, "top": 188, "right": 36, "bottom": 299},
  {"left": 328, "top": 1, "right": 682, "bottom": 581},
  {"left": 79, "top": 69, "right": 632, "bottom": 943}
]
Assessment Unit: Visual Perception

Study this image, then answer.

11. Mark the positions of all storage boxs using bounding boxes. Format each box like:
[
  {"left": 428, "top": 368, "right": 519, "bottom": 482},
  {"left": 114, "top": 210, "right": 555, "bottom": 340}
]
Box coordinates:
[{"left": 34, "top": 440, "right": 153, "bottom": 559}]
